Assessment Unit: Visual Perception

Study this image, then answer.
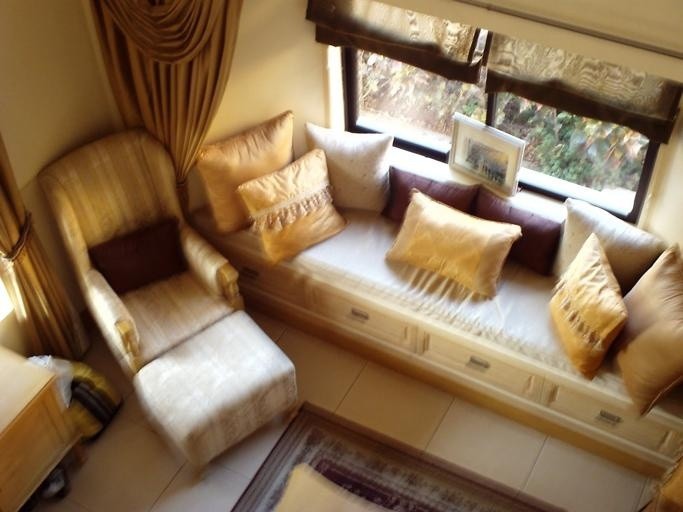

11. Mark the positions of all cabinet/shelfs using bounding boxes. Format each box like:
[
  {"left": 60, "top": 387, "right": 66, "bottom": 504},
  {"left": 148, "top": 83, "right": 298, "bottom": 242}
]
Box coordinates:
[{"left": 1, "top": 348, "right": 87, "bottom": 512}]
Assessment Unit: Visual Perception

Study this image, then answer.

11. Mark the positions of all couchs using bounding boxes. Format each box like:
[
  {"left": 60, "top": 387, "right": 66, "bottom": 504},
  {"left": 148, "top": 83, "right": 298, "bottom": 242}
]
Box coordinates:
[{"left": 201, "top": 196, "right": 683, "bottom": 475}]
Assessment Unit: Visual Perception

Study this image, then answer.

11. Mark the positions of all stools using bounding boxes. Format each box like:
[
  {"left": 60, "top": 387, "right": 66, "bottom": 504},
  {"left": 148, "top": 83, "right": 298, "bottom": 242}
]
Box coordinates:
[{"left": 131, "top": 311, "right": 298, "bottom": 473}]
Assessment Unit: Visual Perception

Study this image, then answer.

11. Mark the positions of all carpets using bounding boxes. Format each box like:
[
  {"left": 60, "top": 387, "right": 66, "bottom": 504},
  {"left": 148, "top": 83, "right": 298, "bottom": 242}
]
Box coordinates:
[{"left": 219, "top": 401, "right": 564, "bottom": 511}]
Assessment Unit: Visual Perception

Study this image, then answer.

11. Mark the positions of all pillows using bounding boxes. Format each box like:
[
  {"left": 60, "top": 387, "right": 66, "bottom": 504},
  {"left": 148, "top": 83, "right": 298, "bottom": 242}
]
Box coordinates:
[
  {"left": 385, "top": 166, "right": 478, "bottom": 228},
  {"left": 303, "top": 125, "right": 393, "bottom": 215},
  {"left": 547, "top": 235, "right": 623, "bottom": 377},
  {"left": 238, "top": 151, "right": 335, "bottom": 262},
  {"left": 199, "top": 111, "right": 294, "bottom": 238},
  {"left": 82, "top": 219, "right": 184, "bottom": 298},
  {"left": 549, "top": 197, "right": 659, "bottom": 289},
  {"left": 615, "top": 246, "right": 683, "bottom": 418},
  {"left": 388, "top": 187, "right": 520, "bottom": 304},
  {"left": 476, "top": 187, "right": 563, "bottom": 280}
]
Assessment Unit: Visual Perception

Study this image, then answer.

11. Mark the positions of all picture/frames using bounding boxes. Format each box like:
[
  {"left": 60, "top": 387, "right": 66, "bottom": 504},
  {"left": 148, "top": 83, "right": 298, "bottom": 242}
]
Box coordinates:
[{"left": 447, "top": 111, "right": 525, "bottom": 197}]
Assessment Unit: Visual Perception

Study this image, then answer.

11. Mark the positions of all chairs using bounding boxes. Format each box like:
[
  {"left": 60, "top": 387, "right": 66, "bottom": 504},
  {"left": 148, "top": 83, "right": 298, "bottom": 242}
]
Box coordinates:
[{"left": 41, "top": 132, "right": 241, "bottom": 371}]
